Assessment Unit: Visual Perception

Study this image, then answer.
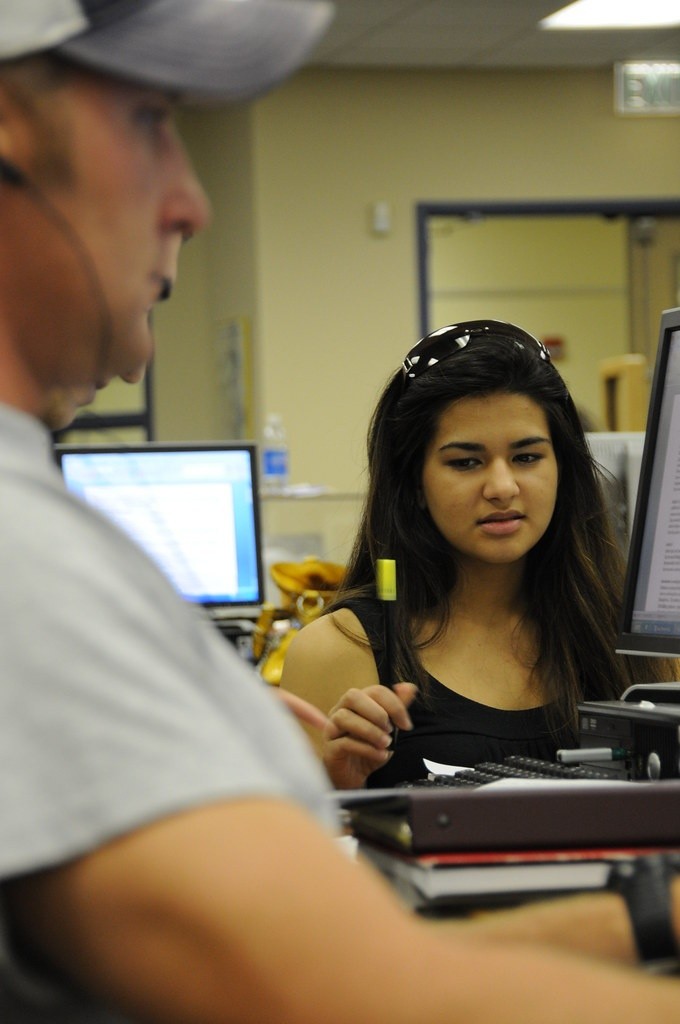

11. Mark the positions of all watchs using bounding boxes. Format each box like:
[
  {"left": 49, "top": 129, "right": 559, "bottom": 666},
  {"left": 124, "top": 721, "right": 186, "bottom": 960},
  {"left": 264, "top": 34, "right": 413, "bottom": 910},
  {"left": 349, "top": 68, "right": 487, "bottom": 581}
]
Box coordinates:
[{"left": 607, "top": 852, "right": 680, "bottom": 963}]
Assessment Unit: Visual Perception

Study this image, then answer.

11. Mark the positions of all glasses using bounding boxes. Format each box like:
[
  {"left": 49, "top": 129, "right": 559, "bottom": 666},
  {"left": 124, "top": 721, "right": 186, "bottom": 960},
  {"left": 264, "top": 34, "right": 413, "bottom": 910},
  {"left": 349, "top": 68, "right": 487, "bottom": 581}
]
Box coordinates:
[{"left": 402, "top": 320, "right": 550, "bottom": 383}]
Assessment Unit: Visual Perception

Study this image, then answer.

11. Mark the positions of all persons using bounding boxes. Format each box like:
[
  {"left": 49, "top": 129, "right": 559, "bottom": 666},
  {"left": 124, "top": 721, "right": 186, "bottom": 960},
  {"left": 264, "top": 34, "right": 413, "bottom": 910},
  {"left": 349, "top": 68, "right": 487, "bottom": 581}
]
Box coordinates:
[
  {"left": 277, "top": 319, "right": 680, "bottom": 792},
  {"left": 0, "top": 0, "right": 680, "bottom": 1024}
]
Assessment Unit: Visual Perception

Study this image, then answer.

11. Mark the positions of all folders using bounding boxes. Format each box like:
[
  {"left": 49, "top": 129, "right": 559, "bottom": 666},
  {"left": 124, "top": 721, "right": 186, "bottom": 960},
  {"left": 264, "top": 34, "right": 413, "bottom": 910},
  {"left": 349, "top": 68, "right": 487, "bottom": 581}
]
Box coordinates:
[{"left": 336, "top": 781, "right": 680, "bottom": 857}]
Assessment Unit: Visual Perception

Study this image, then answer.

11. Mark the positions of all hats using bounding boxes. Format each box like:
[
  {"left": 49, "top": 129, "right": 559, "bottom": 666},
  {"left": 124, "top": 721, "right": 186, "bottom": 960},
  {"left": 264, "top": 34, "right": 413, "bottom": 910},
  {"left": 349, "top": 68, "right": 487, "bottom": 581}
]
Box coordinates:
[{"left": 0, "top": 0, "right": 338, "bottom": 99}]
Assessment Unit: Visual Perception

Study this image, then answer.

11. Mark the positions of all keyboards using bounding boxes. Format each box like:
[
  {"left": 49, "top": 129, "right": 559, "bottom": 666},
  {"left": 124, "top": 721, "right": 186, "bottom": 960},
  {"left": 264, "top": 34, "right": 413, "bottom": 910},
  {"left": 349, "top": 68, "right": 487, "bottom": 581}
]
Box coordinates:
[{"left": 399, "top": 755, "right": 617, "bottom": 791}]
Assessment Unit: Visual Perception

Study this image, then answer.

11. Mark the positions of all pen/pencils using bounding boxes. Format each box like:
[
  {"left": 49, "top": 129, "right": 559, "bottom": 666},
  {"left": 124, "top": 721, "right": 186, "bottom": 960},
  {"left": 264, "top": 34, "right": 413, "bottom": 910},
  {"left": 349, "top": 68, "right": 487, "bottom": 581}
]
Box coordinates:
[
  {"left": 554, "top": 747, "right": 632, "bottom": 764},
  {"left": 376, "top": 557, "right": 400, "bottom": 748}
]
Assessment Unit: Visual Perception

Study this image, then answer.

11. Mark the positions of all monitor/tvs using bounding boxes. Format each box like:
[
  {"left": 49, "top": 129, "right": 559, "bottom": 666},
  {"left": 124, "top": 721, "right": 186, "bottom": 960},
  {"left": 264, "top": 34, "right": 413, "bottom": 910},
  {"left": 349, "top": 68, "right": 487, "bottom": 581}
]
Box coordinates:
[
  {"left": 615, "top": 308, "right": 680, "bottom": 701},
  {"left": 54, "top": 440, "right": 268, "bottom": 619}
]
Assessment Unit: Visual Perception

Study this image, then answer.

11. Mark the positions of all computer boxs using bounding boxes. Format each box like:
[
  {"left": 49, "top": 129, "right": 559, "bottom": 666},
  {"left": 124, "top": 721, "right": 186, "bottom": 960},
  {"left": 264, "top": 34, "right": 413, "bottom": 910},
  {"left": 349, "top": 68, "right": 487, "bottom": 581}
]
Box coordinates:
[{"left": 576, "top": 699, "right": 680, "bottom": 782}]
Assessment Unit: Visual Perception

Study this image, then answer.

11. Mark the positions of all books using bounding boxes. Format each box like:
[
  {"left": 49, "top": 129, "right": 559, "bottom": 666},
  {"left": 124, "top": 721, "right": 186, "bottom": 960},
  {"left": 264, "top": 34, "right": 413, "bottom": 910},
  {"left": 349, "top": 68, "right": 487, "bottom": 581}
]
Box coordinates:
[{"left": 355, "top": 847, "right": 680, "bottom": 918}]
